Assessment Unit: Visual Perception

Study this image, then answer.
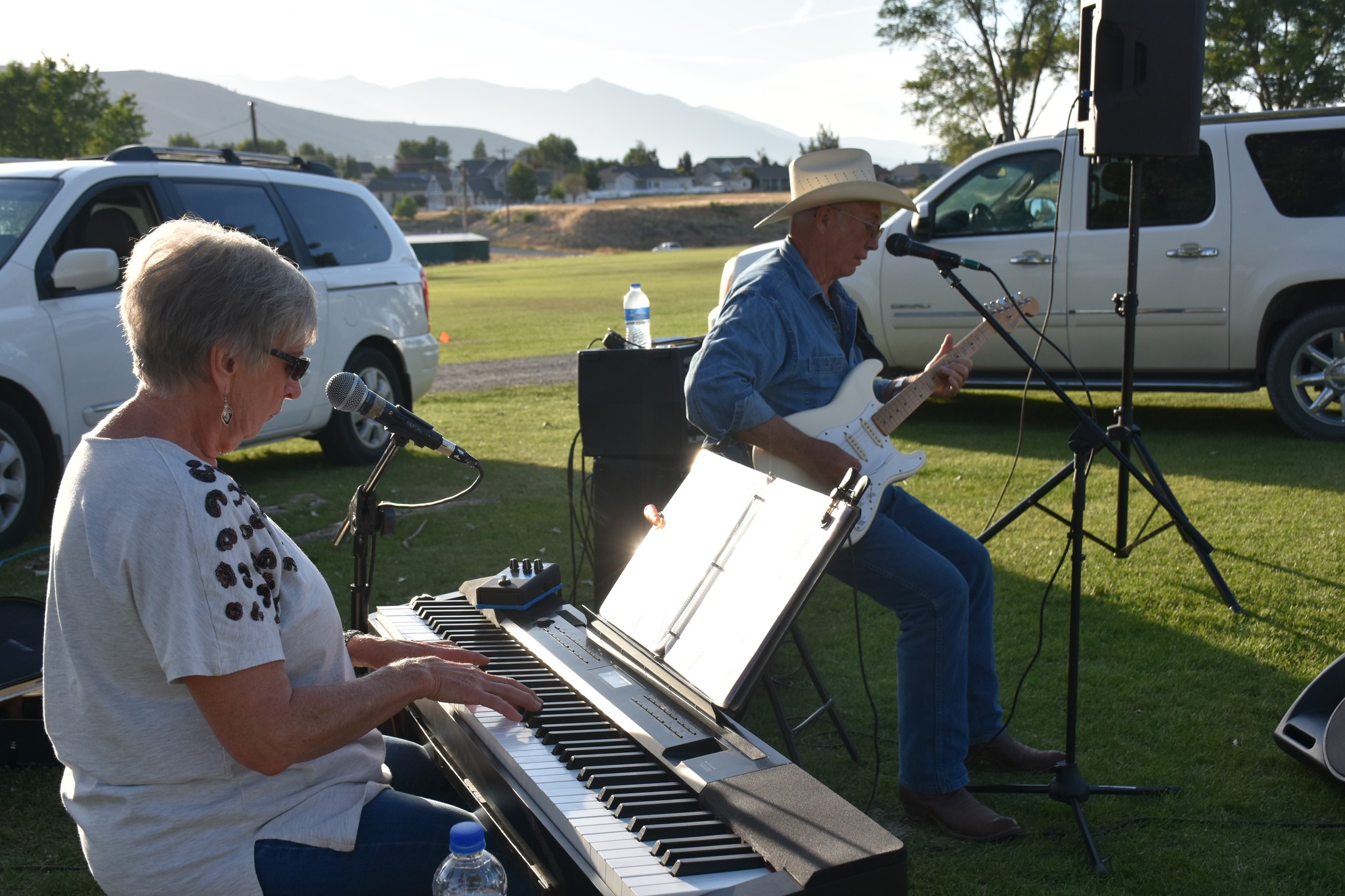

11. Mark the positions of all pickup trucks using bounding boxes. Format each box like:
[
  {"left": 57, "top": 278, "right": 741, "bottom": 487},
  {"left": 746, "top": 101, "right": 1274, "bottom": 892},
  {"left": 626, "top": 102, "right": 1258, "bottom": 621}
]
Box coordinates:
[{"left": 708, "top": 105, "right": 1345, "bottom": 444}]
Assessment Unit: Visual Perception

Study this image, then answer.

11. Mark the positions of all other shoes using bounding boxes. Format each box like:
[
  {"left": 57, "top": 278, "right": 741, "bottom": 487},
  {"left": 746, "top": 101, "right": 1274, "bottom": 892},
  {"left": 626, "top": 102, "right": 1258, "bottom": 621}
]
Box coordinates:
[
  {"left": 966, "top": 730, "right": 1066, "bottom": 771},
  {"left": 897, "top": 782, "right": 1023, "bottom": 841}
]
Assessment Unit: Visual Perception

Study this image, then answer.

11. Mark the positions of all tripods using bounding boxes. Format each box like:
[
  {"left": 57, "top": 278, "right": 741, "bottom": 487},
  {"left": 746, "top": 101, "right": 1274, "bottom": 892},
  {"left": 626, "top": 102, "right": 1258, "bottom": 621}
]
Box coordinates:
[
  {"left": 976, "top": 159, "right": 1241, "bottom": 614},
  {"left": 932, "top": 262, "right": 1181, "bottom": 877}
]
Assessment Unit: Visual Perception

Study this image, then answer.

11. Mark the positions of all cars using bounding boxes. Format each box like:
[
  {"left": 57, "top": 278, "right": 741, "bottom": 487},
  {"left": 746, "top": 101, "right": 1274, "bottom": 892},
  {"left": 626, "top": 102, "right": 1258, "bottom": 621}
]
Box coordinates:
[{"left": 651, "top": 242, "right": 681, "bottom": 253}]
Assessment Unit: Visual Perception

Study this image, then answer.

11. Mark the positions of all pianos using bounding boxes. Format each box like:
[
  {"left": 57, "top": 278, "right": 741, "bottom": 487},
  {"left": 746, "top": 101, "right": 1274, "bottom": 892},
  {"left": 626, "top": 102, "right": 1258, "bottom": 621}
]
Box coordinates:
[{"left": 359, "top": 578, "right": 908, "bottom": 894}]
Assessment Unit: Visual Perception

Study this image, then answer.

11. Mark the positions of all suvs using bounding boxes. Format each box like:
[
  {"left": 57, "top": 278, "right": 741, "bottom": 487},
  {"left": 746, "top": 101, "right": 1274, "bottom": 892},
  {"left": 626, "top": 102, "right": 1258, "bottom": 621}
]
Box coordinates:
[{"left": 1, "top": 143, "right": 438, "bottom": 554}]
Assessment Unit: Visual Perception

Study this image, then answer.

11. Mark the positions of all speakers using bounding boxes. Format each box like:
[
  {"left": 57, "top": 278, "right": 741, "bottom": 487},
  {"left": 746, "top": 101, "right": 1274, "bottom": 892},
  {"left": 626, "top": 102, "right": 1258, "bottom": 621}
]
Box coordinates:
[
  {"left": 1273, "top": 651, "right": 1345, "bottom": 788},
  {"left": 1076, "top": 0, "right": 1208, "bottom": 155},
  {"left": 576, "top": 341, "right": 706, "bottom": 612}
]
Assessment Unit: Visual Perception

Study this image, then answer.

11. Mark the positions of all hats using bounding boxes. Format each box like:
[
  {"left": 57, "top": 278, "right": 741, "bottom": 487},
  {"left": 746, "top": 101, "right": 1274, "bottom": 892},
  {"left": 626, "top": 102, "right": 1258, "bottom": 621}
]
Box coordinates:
[{"left": 752, "top": 147, "right": 919, "bottom": 229}]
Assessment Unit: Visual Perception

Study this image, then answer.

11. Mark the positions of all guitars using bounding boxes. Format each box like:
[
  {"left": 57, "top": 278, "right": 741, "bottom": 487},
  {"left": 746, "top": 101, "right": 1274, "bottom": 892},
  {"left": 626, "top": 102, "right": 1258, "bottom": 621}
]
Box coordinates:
[{"left": 750, "top": 292, "right": 1040, "bottom": 549}]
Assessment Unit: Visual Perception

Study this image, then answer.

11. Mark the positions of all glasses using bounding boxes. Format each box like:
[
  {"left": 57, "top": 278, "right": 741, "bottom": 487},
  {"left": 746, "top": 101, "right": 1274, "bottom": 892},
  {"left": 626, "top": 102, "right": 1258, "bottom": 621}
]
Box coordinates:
[
  {"left": 251, "top": 341, "right": 311, "bottom": 381},
  {"left": 813, "top": 204, "right": 885, "bottom": 240}
]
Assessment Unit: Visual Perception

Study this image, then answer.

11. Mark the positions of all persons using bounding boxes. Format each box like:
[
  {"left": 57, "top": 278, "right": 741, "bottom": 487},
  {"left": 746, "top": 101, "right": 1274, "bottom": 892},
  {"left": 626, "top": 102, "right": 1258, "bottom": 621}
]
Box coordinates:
[
  {"left": 682, "top": 148, "right": 1067, "bottom": 840},
  {"left": 41, "top": 217, "right": 543, "bottom": 896}
]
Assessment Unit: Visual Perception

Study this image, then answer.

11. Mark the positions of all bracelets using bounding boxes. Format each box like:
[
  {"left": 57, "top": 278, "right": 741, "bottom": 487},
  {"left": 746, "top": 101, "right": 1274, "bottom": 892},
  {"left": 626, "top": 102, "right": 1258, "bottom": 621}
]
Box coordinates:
[
  {"left": 344, "top": 630, "right": 364, "bottom": 646},
  {"left": 892, "top": 376, "right": 908, "bottom": 398}
]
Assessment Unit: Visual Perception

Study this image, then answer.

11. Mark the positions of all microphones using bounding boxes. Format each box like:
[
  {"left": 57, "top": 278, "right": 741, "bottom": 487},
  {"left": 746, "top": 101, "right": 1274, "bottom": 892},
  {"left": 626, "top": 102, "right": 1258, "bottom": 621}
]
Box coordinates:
[
  {"left": 326, "top": 371, "right": 479, "bottom": 469},
  {"left": 885, "top": 233, "right": 991, "bottom": 272}
]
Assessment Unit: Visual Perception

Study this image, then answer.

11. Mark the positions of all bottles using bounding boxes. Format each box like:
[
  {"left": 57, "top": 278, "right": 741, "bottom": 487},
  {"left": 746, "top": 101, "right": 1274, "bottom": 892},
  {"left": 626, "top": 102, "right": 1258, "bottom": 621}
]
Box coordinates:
[
  {"left": 623, "top": 283, "right": 652, "bottom": 349},
  {"left": 432, "top": 822, "right": 507, "bottom": 896}
]
